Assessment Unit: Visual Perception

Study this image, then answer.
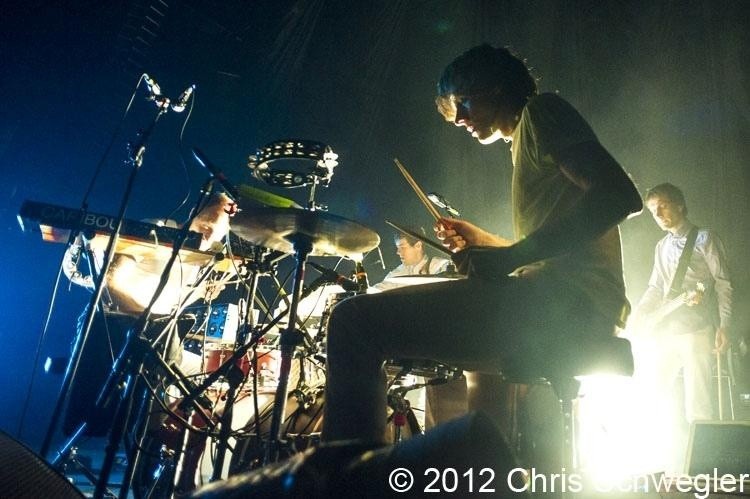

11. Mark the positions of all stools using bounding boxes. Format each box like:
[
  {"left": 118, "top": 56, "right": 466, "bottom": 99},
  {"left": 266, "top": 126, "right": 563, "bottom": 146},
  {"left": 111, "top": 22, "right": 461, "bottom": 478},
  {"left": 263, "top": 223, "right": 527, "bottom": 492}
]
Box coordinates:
[{"left": 501, "top": 334, "right": 633, "bottom": 498}]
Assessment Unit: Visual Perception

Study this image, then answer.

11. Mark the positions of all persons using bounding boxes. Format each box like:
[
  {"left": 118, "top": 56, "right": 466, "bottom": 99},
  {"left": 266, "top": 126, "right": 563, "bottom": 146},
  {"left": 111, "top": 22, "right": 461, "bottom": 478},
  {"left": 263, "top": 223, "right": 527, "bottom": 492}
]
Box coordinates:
[
  {"left": 637, "top": 180, "right": 736, "bottom": 480},
  {"left": 318, "top": 41, "right": 645, "bottom": 446},
  {"left": 104, "top": 190, "right": 240, "bottom": 497},
  {"left": 383, "top": 221, "right": 453, "bottom": 280}
]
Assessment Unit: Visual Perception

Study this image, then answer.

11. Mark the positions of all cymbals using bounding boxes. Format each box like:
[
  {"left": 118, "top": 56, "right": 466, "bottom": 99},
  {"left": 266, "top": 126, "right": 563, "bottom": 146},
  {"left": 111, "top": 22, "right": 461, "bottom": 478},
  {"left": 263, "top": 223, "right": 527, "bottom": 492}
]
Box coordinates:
[
  {"left": 227, "top": 206, "right": 381, "bottom": 257},
  {"left": 385, "top": 269, "right": 467, "bottom": 284}
]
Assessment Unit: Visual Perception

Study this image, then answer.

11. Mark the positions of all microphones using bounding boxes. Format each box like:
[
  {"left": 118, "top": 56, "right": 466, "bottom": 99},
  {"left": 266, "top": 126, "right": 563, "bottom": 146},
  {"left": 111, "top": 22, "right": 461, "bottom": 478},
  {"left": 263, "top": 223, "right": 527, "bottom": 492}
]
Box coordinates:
[
  {"left": 80, "top": 236, "right": 113, "bottom": 309},
  {"left": 426, "top": 189, "right": 462, "bottom": 219},
  {"left": 190, "top": 142, "right": 242, "bottom": 205},
  {"left": 170, "top": 80, "right": 193, "bottom": 113},
  {"left": 143, "top": 72, "right": 171, "bottom": 114},
  {"left": 304, "top": 258, "right": 362, "bottom": 293}
]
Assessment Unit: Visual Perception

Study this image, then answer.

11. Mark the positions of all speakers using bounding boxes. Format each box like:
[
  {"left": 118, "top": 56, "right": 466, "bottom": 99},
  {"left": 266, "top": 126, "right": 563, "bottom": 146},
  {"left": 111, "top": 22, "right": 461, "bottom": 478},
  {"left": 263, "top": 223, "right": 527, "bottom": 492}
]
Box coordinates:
[{"left": 1, "top": 425, "right": 92, "bottom": 497}]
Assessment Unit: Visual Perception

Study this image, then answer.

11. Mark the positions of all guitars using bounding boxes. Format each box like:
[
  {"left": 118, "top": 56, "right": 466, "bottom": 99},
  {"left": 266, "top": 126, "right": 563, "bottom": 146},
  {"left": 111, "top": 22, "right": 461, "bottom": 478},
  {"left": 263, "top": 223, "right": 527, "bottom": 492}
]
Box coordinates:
[{"left": 619, "top": 281, "right": 710, "bottom": 345}]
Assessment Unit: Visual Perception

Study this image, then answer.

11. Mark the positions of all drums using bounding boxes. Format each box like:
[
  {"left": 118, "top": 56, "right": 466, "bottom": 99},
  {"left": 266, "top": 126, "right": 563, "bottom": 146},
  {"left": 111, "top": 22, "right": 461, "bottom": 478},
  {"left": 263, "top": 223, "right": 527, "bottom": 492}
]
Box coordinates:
[
  {"left": 229, "top": 381, "right": 423, "bottom": 478},
  {"left": 311, "top": 291, "right": 458, "bottom": 383},
  {"left": 202, "top": 343, "right": 281, "bottom": 392},
  {"left": 138, "top": 387, "right": 278, "bottom": 497}
]
